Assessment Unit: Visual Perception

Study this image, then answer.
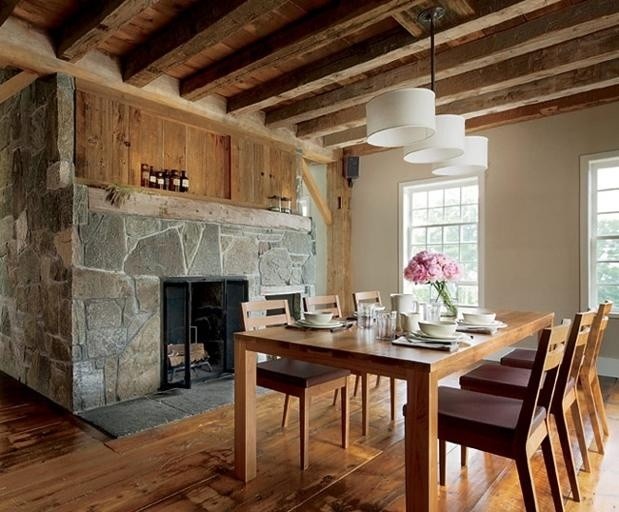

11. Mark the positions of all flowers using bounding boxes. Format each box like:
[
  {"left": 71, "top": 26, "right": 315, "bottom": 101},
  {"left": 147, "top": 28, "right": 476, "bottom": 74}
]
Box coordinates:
[{"left": 404, "top": 250, "right": 462, "bottom": 316}]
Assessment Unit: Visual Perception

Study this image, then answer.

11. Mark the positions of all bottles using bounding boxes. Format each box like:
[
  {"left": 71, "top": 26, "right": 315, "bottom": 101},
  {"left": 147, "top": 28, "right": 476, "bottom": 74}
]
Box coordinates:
[
  {"left": 172, "top": 169, "right": 180, "bottom": 191},
  {"left": 180, "top": 170, "right": 189, "bottom": 192},
  {"left": 157, "top": 172, "right": 164, "bottom": 189},
  {"left": 149, "top": 165, "right": 156, "bottom": 187},
  {"left": 142, "top": 163, "right": 149, "bottom": 188},
  {"left": 164, "top": 168, "right": 171, "bottom": 190}
]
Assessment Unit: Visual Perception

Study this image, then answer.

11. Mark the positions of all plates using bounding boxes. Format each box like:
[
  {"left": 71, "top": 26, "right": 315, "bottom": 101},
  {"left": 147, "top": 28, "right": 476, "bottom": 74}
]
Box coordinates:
[
  {"left": 411, "top": 329, "right": 464, "bottom": 339},
  {"left": 456, "top": 320, "right": 507, "bottom": 328},
  {"left": 295, "top": 319, "right": 343, "bottom": 329}
]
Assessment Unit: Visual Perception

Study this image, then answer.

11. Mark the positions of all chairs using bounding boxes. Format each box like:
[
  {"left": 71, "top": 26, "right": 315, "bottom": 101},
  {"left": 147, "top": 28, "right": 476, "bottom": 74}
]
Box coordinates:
[
  {"left": 303, "top": 296, "right": 395, "bottom": 436},
  {"left": 403, "top": 318, "right": 566, "bottom": 512},
  {"left": 459, "top": 309, "right": 598, "bottom": 502},
  {"left": 353, "top": 291, "right": 406, "bottom": 397},
  {"left": 502, "top": 301, "right": 613, "bottom": 455},
  {"left": 239, "top": 300, "right": 350, "bottom": 471}
]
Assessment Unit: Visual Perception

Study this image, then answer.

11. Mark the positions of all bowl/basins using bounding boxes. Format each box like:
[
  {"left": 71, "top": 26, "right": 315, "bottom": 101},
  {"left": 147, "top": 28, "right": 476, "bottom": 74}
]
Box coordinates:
[
  {"left": 303, "top": 312, "right": 333, "bottom": 323},
  {"left": 417, "top": 321, "right": 456, "bottom": 339},
  {"left": 461, "top": 312, "right": 496, "bottom": 324}
]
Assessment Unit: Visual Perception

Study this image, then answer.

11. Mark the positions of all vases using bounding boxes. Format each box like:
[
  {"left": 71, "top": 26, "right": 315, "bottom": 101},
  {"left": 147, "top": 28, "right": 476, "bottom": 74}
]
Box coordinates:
[{"left": 438, "top": 297, "right": 458, "bottom": 318}]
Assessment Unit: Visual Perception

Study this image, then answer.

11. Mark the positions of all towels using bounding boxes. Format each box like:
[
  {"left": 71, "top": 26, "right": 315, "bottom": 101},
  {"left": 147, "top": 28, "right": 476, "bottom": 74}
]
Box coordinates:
[
  {"left": 392, "top": 336, "right": 460, "bottom": 352},
  {"left": 456, "top": 325, "right": 497, "bottom": 335},
  {"left": 287, "top": 321, "right": 351, "bottom": 330}
]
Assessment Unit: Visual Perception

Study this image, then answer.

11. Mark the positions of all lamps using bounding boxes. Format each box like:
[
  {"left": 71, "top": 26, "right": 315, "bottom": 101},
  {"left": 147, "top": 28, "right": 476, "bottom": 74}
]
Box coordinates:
[{"left": 364, "top": 5, "right": 489, "bottom": 177}]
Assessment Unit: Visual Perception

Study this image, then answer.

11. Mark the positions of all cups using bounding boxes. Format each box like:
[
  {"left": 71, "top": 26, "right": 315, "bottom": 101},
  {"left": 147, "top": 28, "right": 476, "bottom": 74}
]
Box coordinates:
[
  {"left": 426, "top": 302, "right": 444, "bottom": 323},
  {"left": 356, "top": 301, "right": 373, "bottom": 330},
  {"left": 374, "top": 305, "right": 387, "bottom": 333},
  {"left": 280, "top": 196, "right": 291, "bottom": 214},
  {"left": 268, "top": 195, "right": 282, "bottom": 212},
  {"left": 379, "top": 310, "right": 397, "bottom": 342},
  {"left": 401, "top": 312, "right": 418, "bottom": 330}
]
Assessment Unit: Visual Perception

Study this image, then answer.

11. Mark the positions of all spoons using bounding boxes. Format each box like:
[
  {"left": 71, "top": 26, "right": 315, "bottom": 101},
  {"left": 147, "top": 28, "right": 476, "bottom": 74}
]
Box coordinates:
[{"left": 330, "top": 321, "right": 353, "bottom": 333}]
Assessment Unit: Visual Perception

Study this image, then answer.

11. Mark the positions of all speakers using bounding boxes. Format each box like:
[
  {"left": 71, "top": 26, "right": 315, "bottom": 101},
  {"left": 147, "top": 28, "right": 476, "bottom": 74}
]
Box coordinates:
[{"left": 343, "top": 153, "right": 360, "bottom": 179}]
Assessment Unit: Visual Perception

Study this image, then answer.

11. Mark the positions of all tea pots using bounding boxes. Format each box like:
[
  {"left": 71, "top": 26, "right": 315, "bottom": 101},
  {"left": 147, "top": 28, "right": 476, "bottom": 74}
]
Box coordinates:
[
  {"left": 400, "top": 312, "right": 420, "bottom": 334},
  {"left": 390, "top": 293, "right": 419, "bottom": 330}
]
